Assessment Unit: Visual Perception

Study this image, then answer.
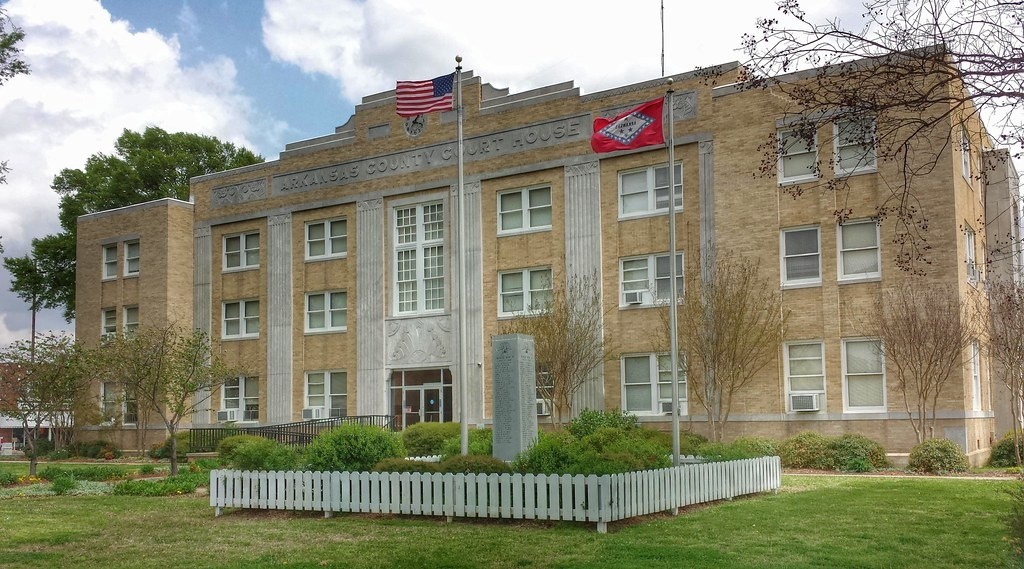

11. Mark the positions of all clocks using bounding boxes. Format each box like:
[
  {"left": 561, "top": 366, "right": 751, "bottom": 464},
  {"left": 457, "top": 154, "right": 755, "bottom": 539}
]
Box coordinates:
[{"left": 403, "top": 114, "right": 426, "bottom": 137}]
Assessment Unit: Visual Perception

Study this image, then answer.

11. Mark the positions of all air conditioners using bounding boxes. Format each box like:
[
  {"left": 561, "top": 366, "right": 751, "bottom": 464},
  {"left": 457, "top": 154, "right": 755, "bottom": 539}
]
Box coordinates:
[
  {"left": 661, "top": 401, "right": 680, "bottom": 413},
  {"left": 537, "top": 399, "right": 552, "bottom": 415},
  {"left": 624, "top": 291, "right": 643, "bottom": 304},
  {"left": 302, "top": 408, "right": 321, "bottom": 420},
  {"left": 217, "top": 409, "right": 238, "bottom": 422},
  {"left": 102, "top": 332, "right": 116, "bottom": 343},
  {"left": 791, "top": 394, "right": 819, "bottom": 411}
]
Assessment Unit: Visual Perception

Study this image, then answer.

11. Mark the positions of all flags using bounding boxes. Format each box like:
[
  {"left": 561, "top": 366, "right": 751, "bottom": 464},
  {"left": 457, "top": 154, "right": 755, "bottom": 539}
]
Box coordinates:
[
  {"left": 395, "top": 72, "right": 456, "bottom": 117},
  {"left": 591, "top": 95, "right": 665, "bottom": 154}
]
